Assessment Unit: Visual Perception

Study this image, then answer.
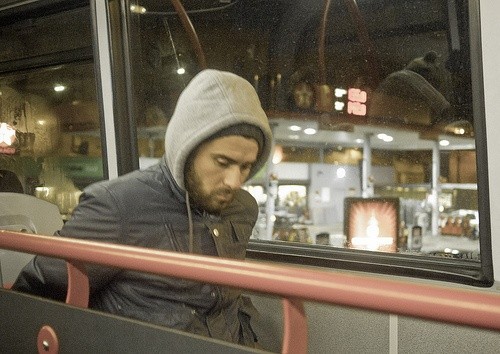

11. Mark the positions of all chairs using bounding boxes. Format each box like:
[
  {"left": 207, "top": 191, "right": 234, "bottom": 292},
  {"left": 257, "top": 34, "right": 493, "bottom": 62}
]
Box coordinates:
[{"left": 0, "top": 192, "right": 64, "bottom": 289}]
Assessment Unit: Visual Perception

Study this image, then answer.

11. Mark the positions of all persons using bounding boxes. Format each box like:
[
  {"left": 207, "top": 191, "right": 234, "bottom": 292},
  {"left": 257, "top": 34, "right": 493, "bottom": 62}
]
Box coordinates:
[{"left": 10, "top": 69, "right": 273, "bottom": 348}]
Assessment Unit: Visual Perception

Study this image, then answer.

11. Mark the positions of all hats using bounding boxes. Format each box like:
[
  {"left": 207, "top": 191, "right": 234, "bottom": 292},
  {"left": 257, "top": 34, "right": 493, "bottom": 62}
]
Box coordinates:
[{"left": 201, "top": 123, "right": 265, "bottom": 160}]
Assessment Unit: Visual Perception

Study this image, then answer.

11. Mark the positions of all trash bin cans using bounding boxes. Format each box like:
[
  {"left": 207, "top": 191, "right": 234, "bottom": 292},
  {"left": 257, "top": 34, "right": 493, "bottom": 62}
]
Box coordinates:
[{"left": 273, "top": 215, "right": 291, "bottom": 241}]
